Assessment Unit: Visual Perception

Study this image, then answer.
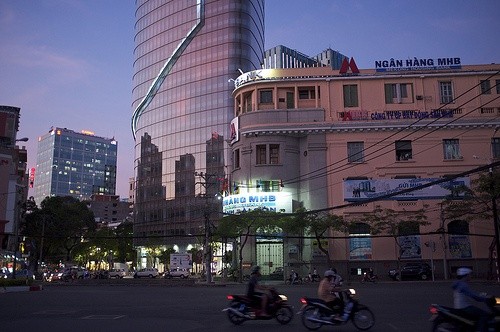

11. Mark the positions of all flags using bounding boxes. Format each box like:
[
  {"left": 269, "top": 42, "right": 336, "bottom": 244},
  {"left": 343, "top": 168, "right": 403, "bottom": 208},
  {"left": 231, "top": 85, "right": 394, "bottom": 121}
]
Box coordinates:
[
  {"left": 349, "top": 57, "right": 360, "bottom": 74},
  {"left": 339, "top": 56, "right": 349, "bottom": 74}
]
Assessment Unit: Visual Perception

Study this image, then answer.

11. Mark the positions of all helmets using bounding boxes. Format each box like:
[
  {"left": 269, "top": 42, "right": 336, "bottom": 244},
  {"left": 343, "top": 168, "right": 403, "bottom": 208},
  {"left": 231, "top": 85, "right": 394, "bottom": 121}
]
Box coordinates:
[
  {"left": 325, "top": 266, "right": 337, "bottom": 277},
  {"left": 251, "top": 266, "right": 263, "bottom": 276}
]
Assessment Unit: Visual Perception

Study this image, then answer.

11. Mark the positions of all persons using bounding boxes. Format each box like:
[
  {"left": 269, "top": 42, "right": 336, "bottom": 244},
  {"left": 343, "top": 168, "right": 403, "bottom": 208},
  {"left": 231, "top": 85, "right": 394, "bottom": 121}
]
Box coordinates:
[
  {"left": 40, "top": 265, "right": 110, "bottom": 284},
  {"left": 453, "top": 268, "right": 497, "bottom": 332},
  {"left": 311, "top": 266, "right": 318, "bottom": 283},
  {"left": 328, "top": 267, "right": 344, "bottom": 302},
  {"left": 317, "top": 270, "right": 350, "bottom": 323},
  {"left": 366, "top": 267, "right": 374, "bottom": 280},
  {"left": 245, "top": 266, "right": 275, "bottom": 317}
]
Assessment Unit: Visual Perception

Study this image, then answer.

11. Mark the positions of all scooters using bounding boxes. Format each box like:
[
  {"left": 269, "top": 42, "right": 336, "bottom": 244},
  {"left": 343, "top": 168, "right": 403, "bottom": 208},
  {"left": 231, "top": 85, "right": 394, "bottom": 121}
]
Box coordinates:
[
  {"left": 285, "top": 272, "right": 305, "bottom": 286},
  {"left": 221, "top": 288, "right": 294, "bottom": 324},
  {"left": 307, "top": 272, "right": 320, "bottom": 282},
  {"left": 360, "top": 273, "right": 377, "bottom": 284}
]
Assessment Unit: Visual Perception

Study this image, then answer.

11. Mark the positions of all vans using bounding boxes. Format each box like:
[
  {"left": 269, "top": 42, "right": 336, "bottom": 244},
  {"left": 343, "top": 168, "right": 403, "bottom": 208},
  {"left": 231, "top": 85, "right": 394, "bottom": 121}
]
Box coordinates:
[
  {"left": 52, "top": 268, "right": 78, "bottom": 280},
  {"left": 136, "top": 268, "right": 158, "bottom": 278}
]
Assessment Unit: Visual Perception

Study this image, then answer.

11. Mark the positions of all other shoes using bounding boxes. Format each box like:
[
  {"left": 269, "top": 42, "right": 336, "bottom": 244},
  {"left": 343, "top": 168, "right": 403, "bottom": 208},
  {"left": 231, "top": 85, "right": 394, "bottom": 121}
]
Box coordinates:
[
  {"left": 261, "top": 313, "right": 270, "bottom": 317},
  {"left": 334, "top": 317, "right": 345, "bottom": 322}
]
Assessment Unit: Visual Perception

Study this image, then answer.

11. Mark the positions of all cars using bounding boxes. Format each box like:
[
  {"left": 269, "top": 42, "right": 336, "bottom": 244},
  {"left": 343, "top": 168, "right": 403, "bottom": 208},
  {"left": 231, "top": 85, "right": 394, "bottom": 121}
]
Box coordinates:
[{"left": 386, "top": 263, "right": 438, "bottom": 280}]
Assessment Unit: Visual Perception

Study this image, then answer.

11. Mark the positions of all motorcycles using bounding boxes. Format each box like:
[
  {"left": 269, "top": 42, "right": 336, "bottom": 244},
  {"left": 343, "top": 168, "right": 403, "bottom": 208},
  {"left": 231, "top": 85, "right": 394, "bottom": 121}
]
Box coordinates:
[
  {"left": 426, "top": 292, "right": 500, "bottom": 332},
  {"left": 296, "top": 286, "right": 375, "bottom": 332}
]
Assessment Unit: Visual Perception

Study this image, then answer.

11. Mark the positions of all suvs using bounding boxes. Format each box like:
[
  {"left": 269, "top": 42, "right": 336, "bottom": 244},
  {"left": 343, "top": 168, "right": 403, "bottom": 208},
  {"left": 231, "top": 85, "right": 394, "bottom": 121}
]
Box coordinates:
[
  {"left": 107, "top": 268, "right": 126, "bottom": 279},
  {"left": 167, "top": 268, "right": 191, "bottom": 278}
]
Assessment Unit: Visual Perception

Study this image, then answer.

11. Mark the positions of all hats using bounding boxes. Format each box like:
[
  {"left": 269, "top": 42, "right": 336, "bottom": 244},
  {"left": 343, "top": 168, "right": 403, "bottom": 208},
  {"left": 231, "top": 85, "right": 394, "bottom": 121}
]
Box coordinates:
[{"left": 456, "top": 268, "right": 472, "bottom": 275}]
